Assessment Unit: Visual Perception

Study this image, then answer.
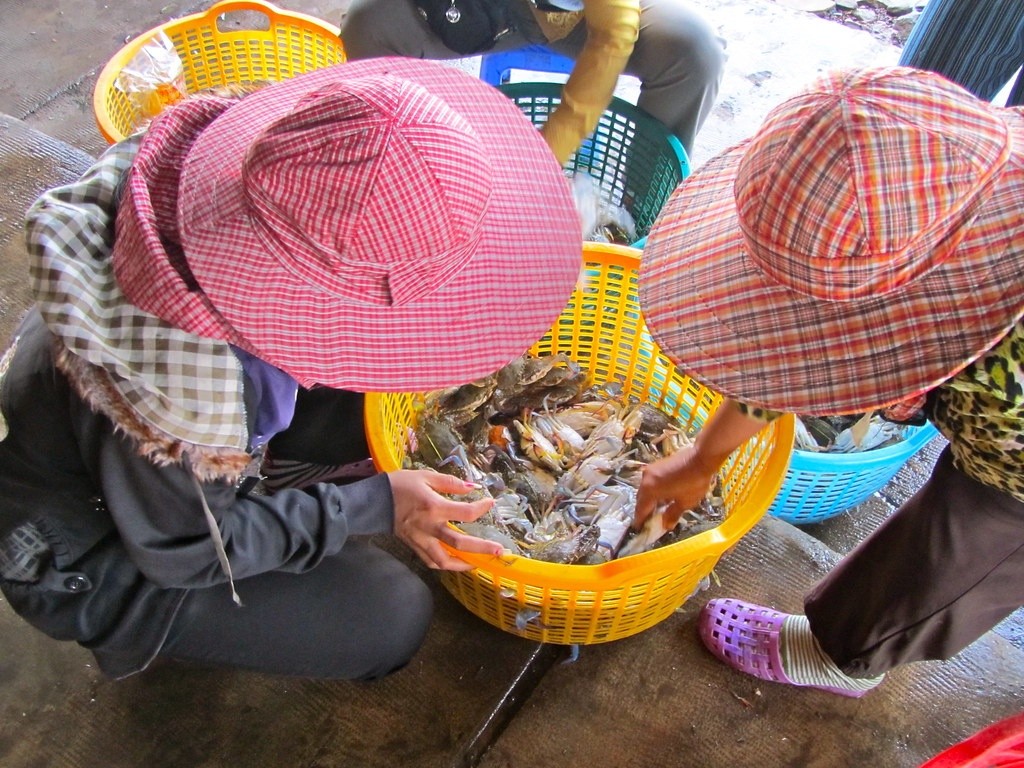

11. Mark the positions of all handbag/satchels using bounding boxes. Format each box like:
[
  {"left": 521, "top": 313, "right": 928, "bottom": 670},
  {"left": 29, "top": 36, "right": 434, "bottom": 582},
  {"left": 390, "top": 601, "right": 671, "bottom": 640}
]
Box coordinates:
[{"left": 415, "top": 0, "right": 499, "bottom": 54}]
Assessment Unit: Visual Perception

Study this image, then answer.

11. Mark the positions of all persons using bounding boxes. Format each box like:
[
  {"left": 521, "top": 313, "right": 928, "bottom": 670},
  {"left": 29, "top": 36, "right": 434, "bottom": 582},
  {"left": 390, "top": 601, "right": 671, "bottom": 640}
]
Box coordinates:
[
  {"left": 0, "top": 53, "right": 577, "bottom": 680},
  {"left": 636, "top": 0, "right": 1024, "bottom": 699},
  {"left": 326, "top": 0, "right": 726, "bottom": 240}
]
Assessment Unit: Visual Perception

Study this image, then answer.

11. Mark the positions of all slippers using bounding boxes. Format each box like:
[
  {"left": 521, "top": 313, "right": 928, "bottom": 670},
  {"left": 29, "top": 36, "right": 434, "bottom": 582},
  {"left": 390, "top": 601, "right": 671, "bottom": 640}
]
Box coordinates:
[{"left": 700, "top": 598, "right": 867, "bottom": 698}]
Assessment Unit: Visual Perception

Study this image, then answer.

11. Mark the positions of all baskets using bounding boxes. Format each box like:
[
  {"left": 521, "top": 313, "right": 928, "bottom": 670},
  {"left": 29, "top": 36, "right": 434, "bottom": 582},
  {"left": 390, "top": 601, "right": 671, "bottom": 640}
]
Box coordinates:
[
  {"left": 363, "top": 242, "right": 797, "bottom": 645},
  {"left": 93, "top": 0, "right": 346, "bottom": 146},
  {"left": 631, "top": 293, "right": 939, "bottom": 524},
  {"left": 485, "top": 85, "right": 690, "bottom": 330}
]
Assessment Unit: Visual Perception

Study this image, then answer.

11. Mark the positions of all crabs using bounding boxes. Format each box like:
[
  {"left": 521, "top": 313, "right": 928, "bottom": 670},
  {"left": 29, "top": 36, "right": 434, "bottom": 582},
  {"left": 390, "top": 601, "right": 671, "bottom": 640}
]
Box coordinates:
[{"left": 402, "top": 353, "right": 726, "bottom": 665}]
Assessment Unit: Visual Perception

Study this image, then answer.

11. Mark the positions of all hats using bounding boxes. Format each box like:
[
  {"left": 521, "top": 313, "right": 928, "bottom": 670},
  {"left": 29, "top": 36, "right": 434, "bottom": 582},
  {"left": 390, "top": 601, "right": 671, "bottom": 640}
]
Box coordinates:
[
  {"left": 176, "top": 56, "right": 584, "bottom": 393},
  {"left": 638, "top": 67, "right": 1024, "bottom": 416}
]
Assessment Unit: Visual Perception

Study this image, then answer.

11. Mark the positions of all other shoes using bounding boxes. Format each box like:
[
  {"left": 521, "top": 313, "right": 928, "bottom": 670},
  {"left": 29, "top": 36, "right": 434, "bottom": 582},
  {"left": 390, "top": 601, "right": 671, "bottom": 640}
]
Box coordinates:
[{"left": 261, "top": 459, "right": 375, "bottom": 495}]
